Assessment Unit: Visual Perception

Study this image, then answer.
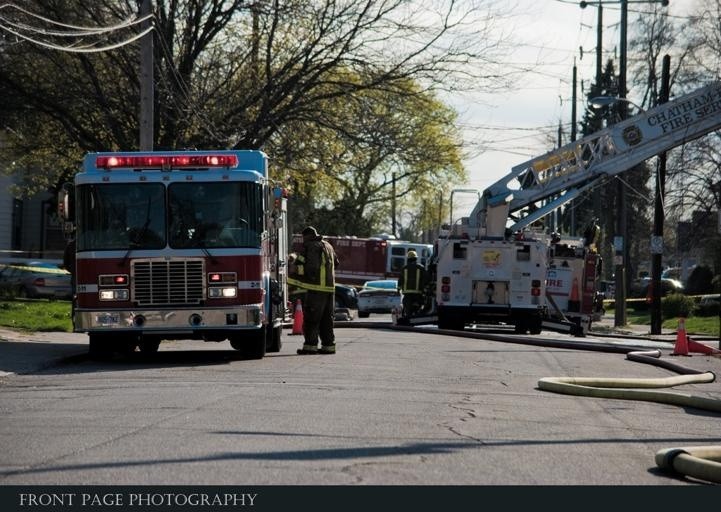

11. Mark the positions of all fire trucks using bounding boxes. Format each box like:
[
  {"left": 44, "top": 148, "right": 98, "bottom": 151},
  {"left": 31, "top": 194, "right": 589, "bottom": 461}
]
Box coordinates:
[
  {"left": 290, "top": 231, "right": 433, "bottom": 292},
  {"left": 59, "top": 149, "right": 289, "bottom": 363}
]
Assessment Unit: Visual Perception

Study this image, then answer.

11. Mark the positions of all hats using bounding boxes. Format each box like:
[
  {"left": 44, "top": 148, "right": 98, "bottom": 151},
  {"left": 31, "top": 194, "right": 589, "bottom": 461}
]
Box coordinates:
[{"left": 301, "top": 226, "right": 316, "bottom": 241}]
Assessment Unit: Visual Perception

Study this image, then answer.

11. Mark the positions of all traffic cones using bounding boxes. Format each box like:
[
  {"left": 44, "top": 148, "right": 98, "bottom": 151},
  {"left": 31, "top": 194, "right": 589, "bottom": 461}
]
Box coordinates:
[
  {"left": 669, "top": 317, "right": 720, "bottom": 356},
  {"left": 292, "top": 299, "right": 304, "bottom": 335}
]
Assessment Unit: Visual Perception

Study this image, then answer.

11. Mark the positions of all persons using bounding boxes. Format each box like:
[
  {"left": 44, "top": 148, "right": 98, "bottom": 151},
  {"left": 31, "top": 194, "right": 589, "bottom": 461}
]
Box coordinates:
[
  {"left": 63, "top": 238, "right": 88, "bottom": 332},
  {"left": 291, "top": 225, "right": 341, "bottom": 355},
  {"left": 397, "top": 251, "right": 429, "bottom": 320}
]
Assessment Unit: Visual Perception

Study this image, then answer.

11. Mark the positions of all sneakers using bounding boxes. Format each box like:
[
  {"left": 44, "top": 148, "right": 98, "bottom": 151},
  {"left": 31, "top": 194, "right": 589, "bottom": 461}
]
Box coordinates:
[{"left": 296, "top": 349, "right": 335, "bottom": 355}]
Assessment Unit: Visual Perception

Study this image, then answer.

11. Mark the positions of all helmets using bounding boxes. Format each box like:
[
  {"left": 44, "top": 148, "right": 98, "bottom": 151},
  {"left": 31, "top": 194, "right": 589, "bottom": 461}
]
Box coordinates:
[{"left": 408, "top": 250, "right": 419, "bottom": 258}]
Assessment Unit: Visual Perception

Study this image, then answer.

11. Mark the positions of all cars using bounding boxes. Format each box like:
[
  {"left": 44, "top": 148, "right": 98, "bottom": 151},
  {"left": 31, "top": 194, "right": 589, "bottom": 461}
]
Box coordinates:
[
  {"left": 358, "top": 280, "right": 405, "bottom": 318},
  {"left": 0, "top": 259, "right": 71, "bottom": 299},
  {"left": 335, "top": 281, "right": 359, "bottom": 308}
]
